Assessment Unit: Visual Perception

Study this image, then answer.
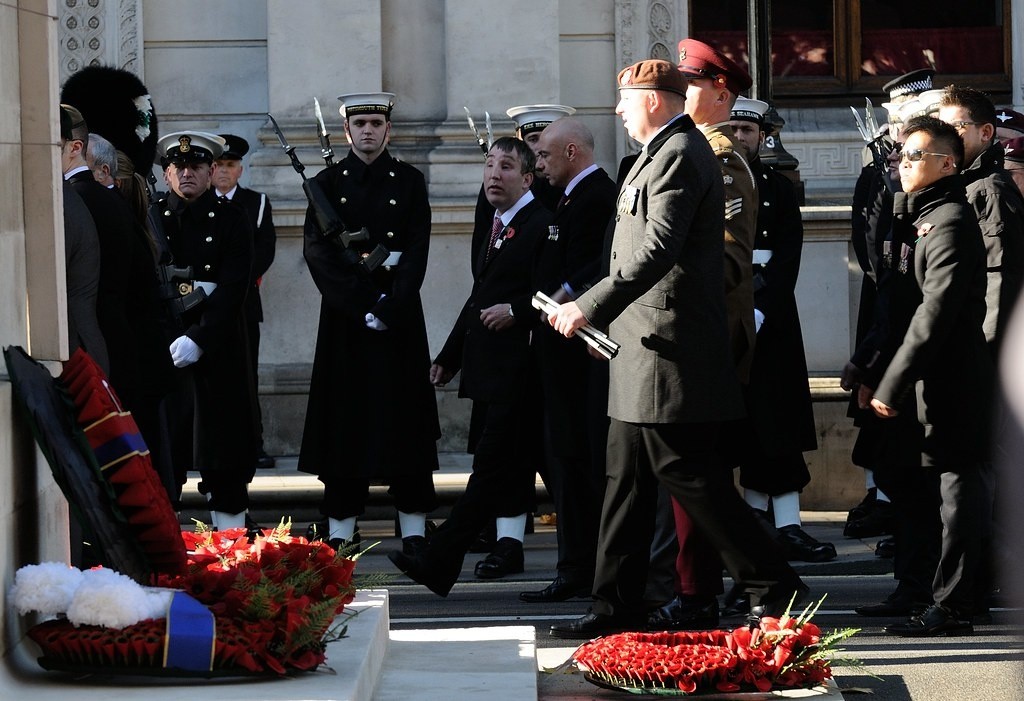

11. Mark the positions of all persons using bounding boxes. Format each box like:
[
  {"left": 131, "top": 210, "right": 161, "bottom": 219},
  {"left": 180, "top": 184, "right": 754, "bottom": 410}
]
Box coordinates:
[
  {"left": 57, "top": 37, "right": 1024, "bottom": 640},
  {"left": 301, "top": 91, "right": 442, "bottom": 561},
  {"left": 547, "top": 59, "right": 811, "bottom": 640}
]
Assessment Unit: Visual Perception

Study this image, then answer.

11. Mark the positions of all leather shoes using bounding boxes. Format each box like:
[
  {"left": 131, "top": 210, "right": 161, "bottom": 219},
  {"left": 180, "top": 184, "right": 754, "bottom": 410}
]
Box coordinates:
[
  {"left": 474, "top": 540, "right": 526, "bottom": 577},
  {"left": 777, "top": 523, "right": 838, "bottom": 562},
  {"left": 881, "top": 601, "right": 976, "bottom": 637}
]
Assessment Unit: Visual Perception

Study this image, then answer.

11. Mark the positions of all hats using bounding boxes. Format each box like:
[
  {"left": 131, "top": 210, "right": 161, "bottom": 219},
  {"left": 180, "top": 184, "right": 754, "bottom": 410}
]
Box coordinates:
[
  {"left": 154, "top": 130, "right": 227, "bottom": 171},
  {"left": 730, "top": 94, "right": 770, "bottom": 125},
  {"left": 617, "top": 60, "right": 689, "bottom": 100},
  {"left": 995, "top": 109, "right": 1024, "bottom": 133},
  {"left": 1001, "top": 136, "right": 1024, "bottom": 163},
  {"left": 674, "top": 39, "right": 754, "bottom": 96},
  {"left": 505, "top": 104, "right": 576, "bottom": 139},
  {"left": 215, "top": 134, "right": 250, "bottom": 161},
  {"left": 336, "top": 92, "right": 397, "bottom": 119},
  {"left": 882, "top": 67, "right": 948, "bottom": 119}
]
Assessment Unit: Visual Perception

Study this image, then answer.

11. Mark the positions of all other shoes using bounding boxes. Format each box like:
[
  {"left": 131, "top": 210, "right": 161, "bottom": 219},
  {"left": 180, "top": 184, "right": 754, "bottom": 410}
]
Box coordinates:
[
  {"left": 854, "top": 582, "right": 934, "bottom": 613},
  {"left": 647, "top": 593, "right": 720, "bottom": 629},
  {"left": 721, "top": 570, "right": 812, "bottom": 627},
  {"left": 842, "top": 492, "right": 900, "bottom": 553},
  {"left": 517, "top": 573, "right": 595, "bottom": 603},
  {"left": 548, "top": 611, "right": 650, "bottom": 639},
  {"left": 387, "top": 543, "right": 461, "bottom": 595}
]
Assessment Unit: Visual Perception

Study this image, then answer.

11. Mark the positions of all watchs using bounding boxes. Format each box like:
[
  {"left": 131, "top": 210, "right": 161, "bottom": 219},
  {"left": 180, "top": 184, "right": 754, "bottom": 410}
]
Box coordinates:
[{"left": 508, "top": 305, "right": 514, "bottom": 319}]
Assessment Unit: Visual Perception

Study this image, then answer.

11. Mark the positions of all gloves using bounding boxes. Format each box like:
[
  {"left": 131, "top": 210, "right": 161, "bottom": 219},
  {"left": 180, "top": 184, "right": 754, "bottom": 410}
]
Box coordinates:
[
  {"left": 169, "top": 335, "right": 202, "bottom": 368},
  {"left": 365, "top": 313, "right": 386, "bottom": 331},
  {"left": 754, "top": 309, "right": 764, "bottom": 333}
]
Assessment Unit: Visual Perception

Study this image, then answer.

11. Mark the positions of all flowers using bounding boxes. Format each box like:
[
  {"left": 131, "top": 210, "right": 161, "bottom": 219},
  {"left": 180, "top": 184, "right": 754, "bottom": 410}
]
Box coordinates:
[
  {"left": 8, "top": 516, "right": 409, "bottom": 678},
  {"left": 571, "top": 591, "right": 885, "bottom": 701}
]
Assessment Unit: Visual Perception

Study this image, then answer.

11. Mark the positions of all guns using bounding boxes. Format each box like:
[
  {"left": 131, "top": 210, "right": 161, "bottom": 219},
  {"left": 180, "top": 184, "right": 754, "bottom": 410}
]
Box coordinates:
[
  {"left": 461, "top": 103, "right": 496, "bottom": 157},
  {"left": 851, "top": 96, "right": 897, "bottom": 195},
  {"left": 312, "top": 96, "right": 335, "bottom": 165},
  {"left": 156, "top": 260, "right": 207, "bottom": 315},
  {"left": 267, "top": 112, "right": 371, "bottom": 271}
]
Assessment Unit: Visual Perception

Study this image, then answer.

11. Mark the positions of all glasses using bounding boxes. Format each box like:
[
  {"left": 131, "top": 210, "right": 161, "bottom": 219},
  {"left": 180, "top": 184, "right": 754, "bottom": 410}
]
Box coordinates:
[
  {"left": 947, "top": 121, "right": 989, "bottom": 130},
  {"left": 898, "top": 149, "right": 957, "bottom": 167}
]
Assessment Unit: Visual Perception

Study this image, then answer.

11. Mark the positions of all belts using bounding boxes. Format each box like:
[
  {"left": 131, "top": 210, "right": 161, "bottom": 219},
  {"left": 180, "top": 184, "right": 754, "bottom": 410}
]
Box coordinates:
[
  {"left": 381, "top": 252, "right": 402, "bottom": 266},
  {"left": 752, "top": 249, "right": 772, "bottom": 264},
  {"left": 177, "top": 280, "right": 218, "bottom": 296}
]
